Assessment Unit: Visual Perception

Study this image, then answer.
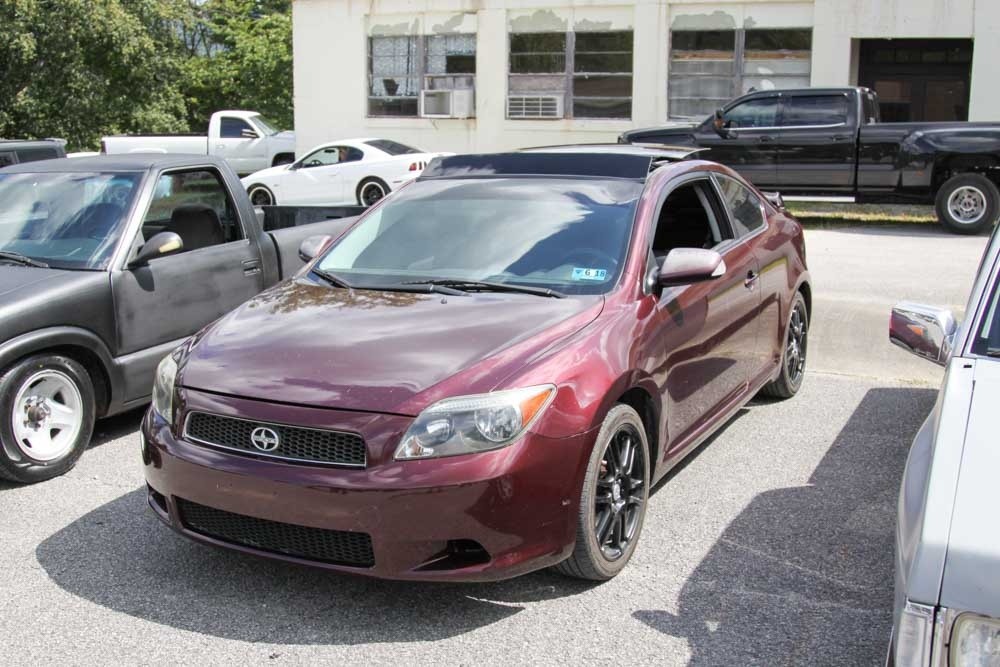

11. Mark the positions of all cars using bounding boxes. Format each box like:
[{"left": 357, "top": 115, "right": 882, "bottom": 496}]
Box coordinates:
[
  {"left": 886, "top": 217, "right": 1000, "bottom": 667},
  {"left": 139, "top": 141, "right": 814, "bottom": 583},
  {"left": 239, "top": 136, "right": 460, "bottom": 207},
  {"left": 0, "top": 136, "right": 174, "bottom": 225}
]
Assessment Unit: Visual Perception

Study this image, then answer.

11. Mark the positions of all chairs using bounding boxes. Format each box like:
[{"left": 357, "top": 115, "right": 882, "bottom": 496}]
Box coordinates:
[
  {"left": 70, "top": 205, "right": 122, "bottom": 239},
  {"left": 168, "top": 207, "right": 222, "bottom": 250}
]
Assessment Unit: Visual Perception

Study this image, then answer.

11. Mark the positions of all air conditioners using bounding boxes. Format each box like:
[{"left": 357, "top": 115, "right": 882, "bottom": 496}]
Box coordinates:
[{"left": 506, "top": 92, "right": 565, "bottom": 118}]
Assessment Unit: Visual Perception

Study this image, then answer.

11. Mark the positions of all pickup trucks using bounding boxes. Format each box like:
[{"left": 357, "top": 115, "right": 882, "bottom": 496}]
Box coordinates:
[
  {"left": 616, "top": 84, "right": 1000, "bottom": 235},
  {"left": 0, "top": 149, "right": 377, "bottom": 484},
  {"left": 98, "top": 109, "right": 295, "bottom": 178}
]
